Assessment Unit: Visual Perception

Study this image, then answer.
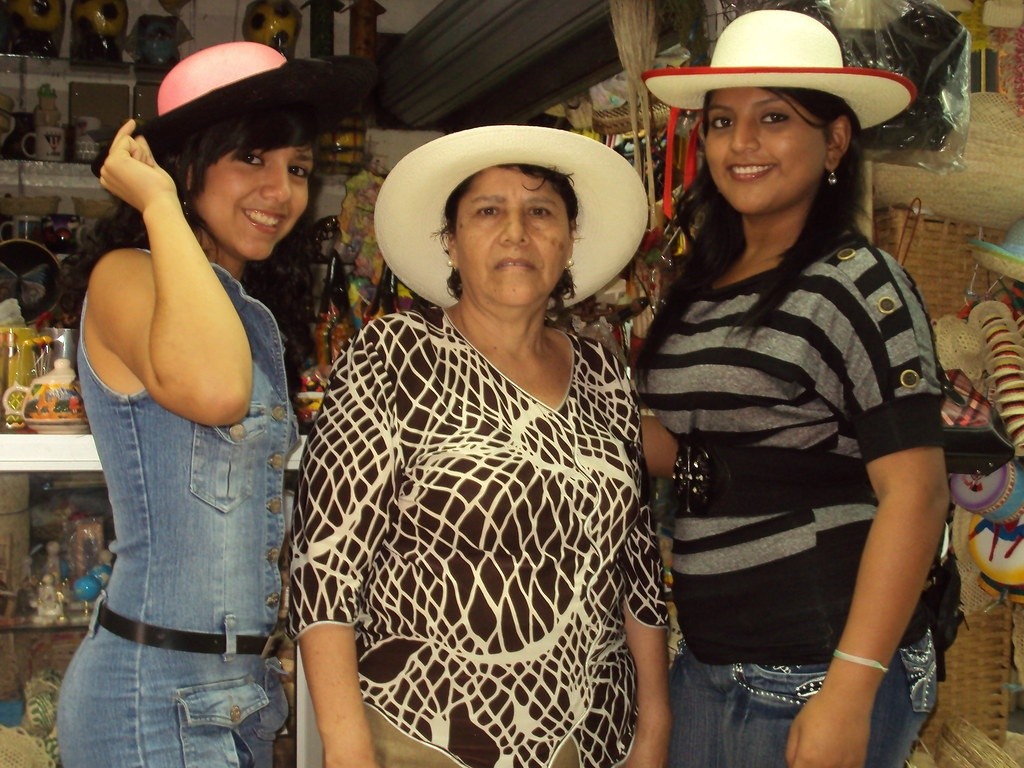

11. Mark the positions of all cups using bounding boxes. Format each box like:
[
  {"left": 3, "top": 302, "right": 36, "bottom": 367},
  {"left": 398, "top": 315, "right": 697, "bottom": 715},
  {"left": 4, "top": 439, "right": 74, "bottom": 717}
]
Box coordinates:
[
  {"left": 0, "top": 92, "right": 16, "bottom": 154},
  {"left": 75, "top": 116, "right": 102, "bottom": 161},
  {"left": 0, "top": 215, "right": 41, "bottom": 242},
  {"left": 21, "top": 127, "right": 65, "bottom": 162}
]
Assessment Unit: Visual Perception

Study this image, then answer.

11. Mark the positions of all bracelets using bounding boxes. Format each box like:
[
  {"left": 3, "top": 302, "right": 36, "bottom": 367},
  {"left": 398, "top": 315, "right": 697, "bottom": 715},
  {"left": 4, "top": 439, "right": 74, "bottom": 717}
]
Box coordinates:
[{"left": 833, "top": 650, "right": 893, "bottom": 672}]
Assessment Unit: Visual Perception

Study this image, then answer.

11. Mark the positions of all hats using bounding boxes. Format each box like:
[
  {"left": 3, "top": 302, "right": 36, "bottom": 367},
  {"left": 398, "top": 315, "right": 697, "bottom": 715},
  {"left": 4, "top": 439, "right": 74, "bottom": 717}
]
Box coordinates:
[
  {"left": 89, "top": 41, "right": 377, "bottom": 180},
  {"left": 967, "top": 215, "right": 1024, "bottom": 283},
  {"left": 372, "top": 124, "right": 649, "bottom": 308},
  {"left": 935, "top": 315, "right": 987, "bottom": 382},
  {"left": 968, "top": 300, "right": 1024, "bottom": 456},
  {"left": 640, "top": 8, "right": 918, "bottom": 129}
]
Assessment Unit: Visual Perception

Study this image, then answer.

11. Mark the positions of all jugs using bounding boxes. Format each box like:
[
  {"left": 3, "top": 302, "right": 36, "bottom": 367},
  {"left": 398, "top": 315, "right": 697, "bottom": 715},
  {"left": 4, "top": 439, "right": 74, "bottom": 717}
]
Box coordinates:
[{"left": 2, "top": 359, "right": 90, "bottom": 434}]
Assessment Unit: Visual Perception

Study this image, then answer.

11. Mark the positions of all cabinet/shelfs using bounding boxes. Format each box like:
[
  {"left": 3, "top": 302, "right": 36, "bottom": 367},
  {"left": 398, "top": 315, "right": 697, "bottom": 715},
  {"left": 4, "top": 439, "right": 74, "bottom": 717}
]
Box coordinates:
[{"left": 0, "top": 1, "right": 383, "bottom": 768}]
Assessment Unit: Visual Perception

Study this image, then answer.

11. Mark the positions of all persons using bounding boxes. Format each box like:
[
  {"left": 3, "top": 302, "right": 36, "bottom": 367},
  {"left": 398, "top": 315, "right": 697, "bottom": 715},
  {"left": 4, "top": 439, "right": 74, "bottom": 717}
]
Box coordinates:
[
  {"left": 632, "top": 9, "right": 951, "bottom": 768},
  {"left": 292, "top": 125, "right": 671, "bottom": 768},
  {"left": 59, "top": 44, "right": 385, "bottom": 768}
]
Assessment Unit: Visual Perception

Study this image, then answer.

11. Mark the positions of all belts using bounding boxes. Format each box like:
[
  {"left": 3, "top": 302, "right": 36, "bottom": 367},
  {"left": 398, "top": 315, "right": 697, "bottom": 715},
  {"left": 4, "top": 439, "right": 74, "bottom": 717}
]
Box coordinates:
[{"left": 94, "top": 602, "right": 282, "bottom": 656}]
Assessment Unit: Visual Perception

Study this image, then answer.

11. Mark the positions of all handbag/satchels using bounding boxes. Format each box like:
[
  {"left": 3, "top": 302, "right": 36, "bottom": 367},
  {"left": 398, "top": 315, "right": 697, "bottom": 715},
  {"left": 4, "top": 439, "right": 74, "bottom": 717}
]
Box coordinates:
[{"left": 935, "top": 355, "right": 1014, "bottom": 478}]
